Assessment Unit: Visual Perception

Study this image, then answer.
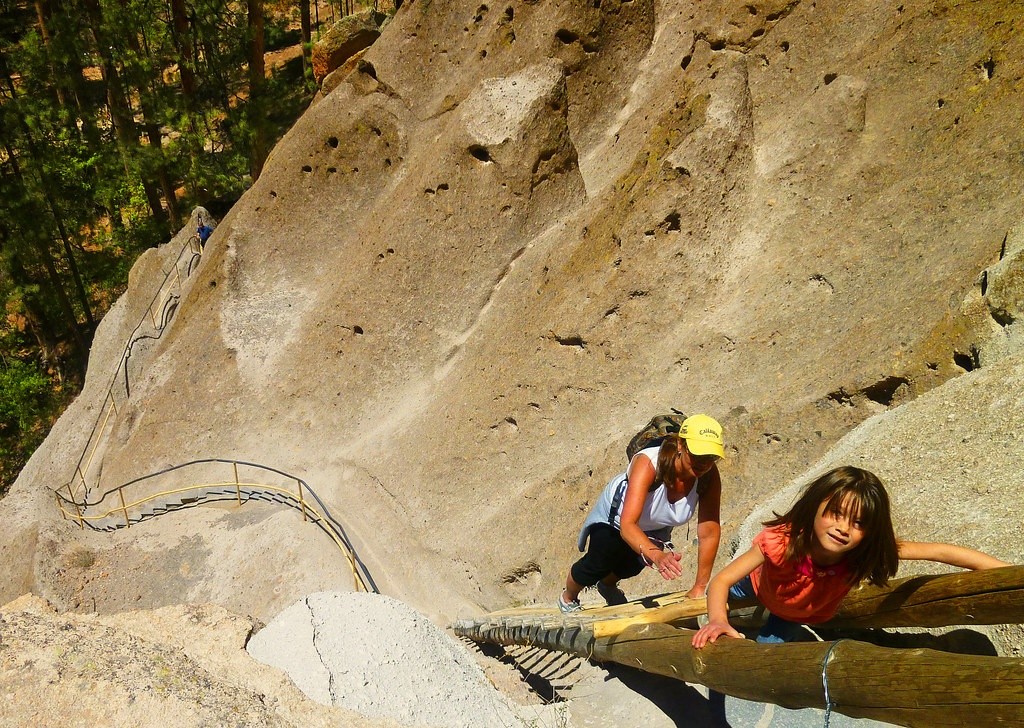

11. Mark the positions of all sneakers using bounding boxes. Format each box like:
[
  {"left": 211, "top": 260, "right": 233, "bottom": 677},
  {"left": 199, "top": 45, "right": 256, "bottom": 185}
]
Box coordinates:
[
  {"left": 557, "top": 588, "right": 585, "bottom": 613},
  {"left": 597, "top": 582, "right": 628, "bottom": 606}
]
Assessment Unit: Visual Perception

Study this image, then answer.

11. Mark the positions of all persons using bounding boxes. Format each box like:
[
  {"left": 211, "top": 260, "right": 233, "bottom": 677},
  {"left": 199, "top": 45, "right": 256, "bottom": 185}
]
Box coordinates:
[
  {"left": 690, "top": 466, "right": 1013, "bottom": 651},
  {"left": 556, "top": 413, "right": 722, "bottom": 615},
  {"left": 196, "top": 221, "right": 215, "bottom": 248}
]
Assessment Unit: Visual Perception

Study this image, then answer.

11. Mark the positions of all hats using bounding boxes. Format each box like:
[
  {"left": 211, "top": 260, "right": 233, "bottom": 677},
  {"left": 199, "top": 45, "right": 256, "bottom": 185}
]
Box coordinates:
[{"left": 679, "top": 414, "right": 726, "bottom": 460}]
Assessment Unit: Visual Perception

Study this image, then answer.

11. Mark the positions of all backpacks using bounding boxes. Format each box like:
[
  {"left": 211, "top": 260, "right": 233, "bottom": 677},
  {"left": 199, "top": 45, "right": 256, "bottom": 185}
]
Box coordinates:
[{"left": 626, "top": 408, "right": 708, "bottom": 497}]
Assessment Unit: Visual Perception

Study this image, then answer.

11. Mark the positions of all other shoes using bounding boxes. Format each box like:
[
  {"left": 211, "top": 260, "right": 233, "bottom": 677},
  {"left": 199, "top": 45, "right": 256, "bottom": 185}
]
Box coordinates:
[{"left": 697, "top": 613, "right": 708, "bottom": 628}]
